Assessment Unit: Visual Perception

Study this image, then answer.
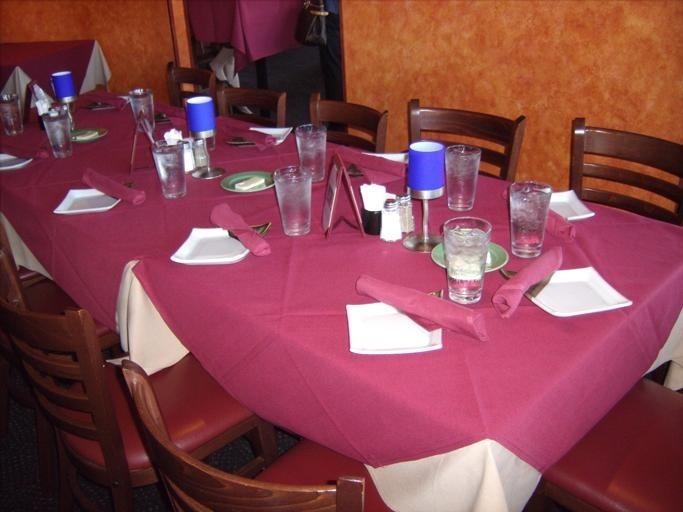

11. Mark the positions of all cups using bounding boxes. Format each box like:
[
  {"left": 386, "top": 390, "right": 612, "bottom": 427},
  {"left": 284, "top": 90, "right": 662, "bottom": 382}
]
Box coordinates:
[
  {"left": 442, "top": 216, "right": 492, "bottom": 304},
  {"left": 128, "top": 88, "right": 155, "bottom": 133},
  {"left": 1, "top": 91, "right": 24, "bottom": 135},
  {"left": 509, "top": 180, "right": 552, "bottom": 258},
  {"left": 272, "top": 165, "right": 313, "bottom": 236},
  {"left": 295, "top": 123, "right": 327, "bottom": 182},
  {"left": 361, "top": 208, "right": 382, "bottom": 234},
  {"left": 41, "top": 109, "right": 73, "bottom": 159},
  {"left": 152, "top": 139, "right": 187, "bottom": 199},
  {"left": 445, "top": 144, "right": 481, "bottom": 211}
]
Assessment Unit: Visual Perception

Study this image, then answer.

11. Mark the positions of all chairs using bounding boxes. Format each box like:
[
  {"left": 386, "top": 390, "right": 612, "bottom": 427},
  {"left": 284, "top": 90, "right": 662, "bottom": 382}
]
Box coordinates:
[{"left": 524, "top": 378, "right": 682, "bottom": 511}]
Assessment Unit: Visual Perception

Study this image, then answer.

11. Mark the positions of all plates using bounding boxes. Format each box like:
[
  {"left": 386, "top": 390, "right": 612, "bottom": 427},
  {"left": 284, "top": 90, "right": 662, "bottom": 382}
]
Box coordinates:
[
  {"left": 225, "top": 127, "right": 293, "bottom": 147},
  {"left": 168, "top": 223, "right": 270, "bottom": 265},
  {"left": 154, "top": 112, "right": 170, "bottom": 123},
  {"left": 361, "top": 151, "right": 408, "bottom": 163},
  {"left": 52, "top": 182, "right": 136, "bottom": 215},
  {"left": 70, "top": 128, "right": 108, "bottom": 143},
  {"left": 431, "top": 241, "right": 509, "bottom": 273},
  {"left": 549, "top": 188, "right": 596, "bottom": 222},
  {"left": 345, "top": 289, "right": 443, "bottom": 355},
  {"left": 501, "top": 265, "right": 633, "bottom": 317},
  {"left": 219, "top": 170, "right": 275, "bottom": 193},
  {"left": 0, "top": 153, "right": 33, "bottom": 171},
  {"left": 83, "top": 95, "right": 129, "bottom": 110}
]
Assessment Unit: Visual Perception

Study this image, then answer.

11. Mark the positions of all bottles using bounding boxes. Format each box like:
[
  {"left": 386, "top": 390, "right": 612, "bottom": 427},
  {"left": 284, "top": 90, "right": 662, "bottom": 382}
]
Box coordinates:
[
  {"left": 182, "top": 141, "right": 196, "bottom": 172},
  {"left": 193, "top": 138, "right": 208, "bottom": 167},
  {"left": 397, "top": 193, "right": 415, "bottom": 234},
  {"left": 379, "top": 199, "right": 402, "bottom": 242}
]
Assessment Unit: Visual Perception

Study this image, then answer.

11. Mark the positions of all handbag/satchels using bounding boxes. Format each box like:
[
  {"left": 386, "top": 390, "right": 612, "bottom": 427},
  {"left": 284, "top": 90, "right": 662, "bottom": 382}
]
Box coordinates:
[{"left": 294, "top": 0, "right": 329, "bottom": 46}]
